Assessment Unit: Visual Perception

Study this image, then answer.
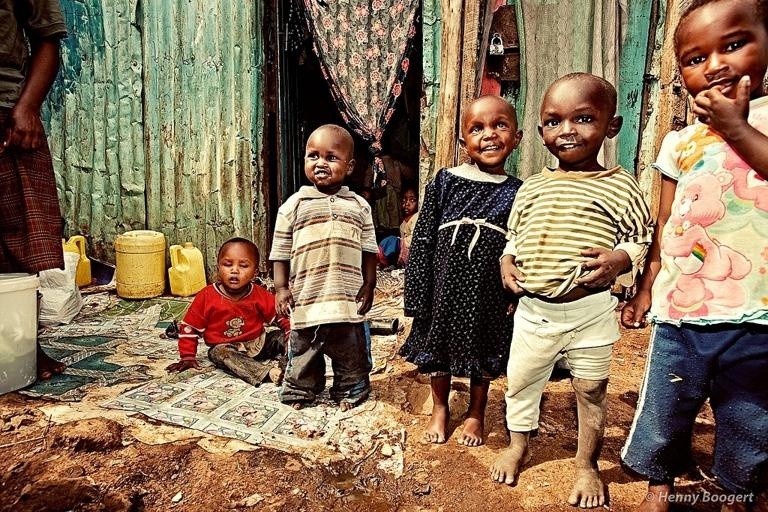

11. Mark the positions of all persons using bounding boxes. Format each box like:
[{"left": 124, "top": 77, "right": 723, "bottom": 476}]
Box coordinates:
[
  {"left": 360, "top": 140, "right": 415, "bottom": 231},
  {"left": 615, "top": 2, "right": 766, "bottom": 511},
  {"left": 0, "top": 0, "right": 67, "bottom": 379},
  {"left": 268, "top": 125, "right": 378, "bottom": 410},
  {"left": 163, "top": 237, "right": 292, "bottom": 386},
  {"left": 487, "top": 73, "right": 654, "bottom": 507},
  {"left": 375, "top": 185, "right": 423, "bottom": 271},
  {"left": 397, "top": 95, "right": 524, "bottom": 447}
]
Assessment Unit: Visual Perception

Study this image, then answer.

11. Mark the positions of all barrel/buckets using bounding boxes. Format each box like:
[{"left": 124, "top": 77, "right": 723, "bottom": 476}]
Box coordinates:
[
  {"left": 0, "top": 273, "right": 40, "bottom": 395},
  {"left": 114, "top": 230, "right": 165, "bottom": 299}
]
